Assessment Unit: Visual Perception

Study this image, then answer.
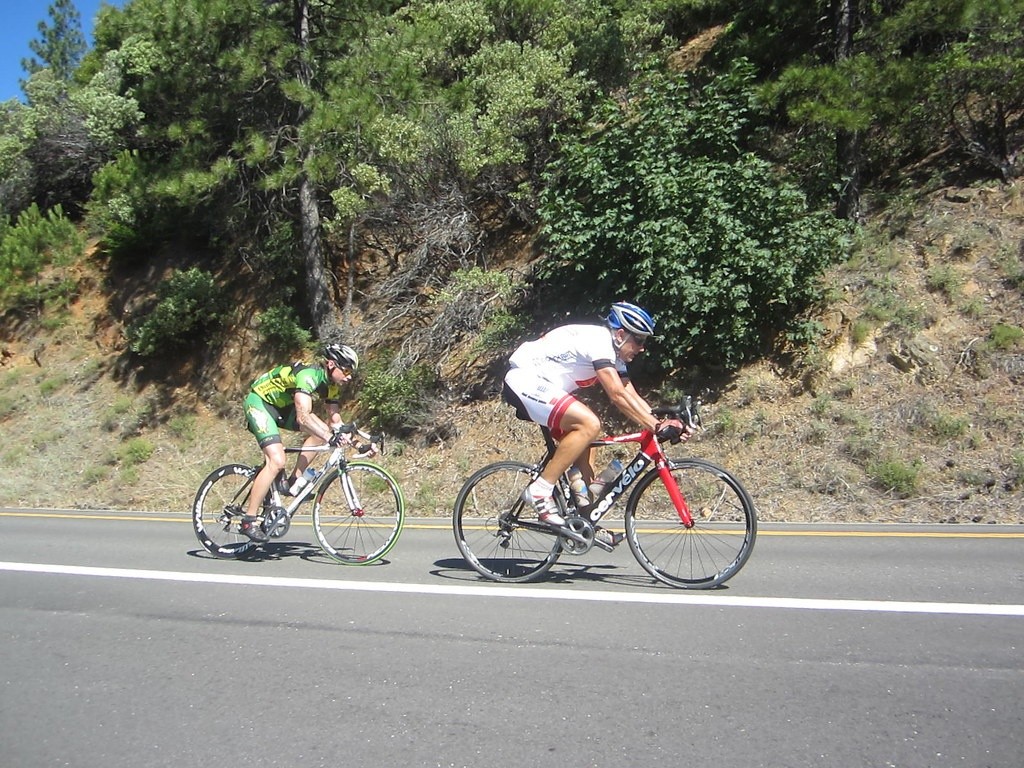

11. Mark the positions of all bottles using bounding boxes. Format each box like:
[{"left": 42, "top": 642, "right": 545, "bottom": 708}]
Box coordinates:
[
  {"left": 588, "top": 457, "right": 623, "bottom": 496},
  {"left": 289, "top": 467, "right": 317, "bottom": 497},
  {"left": 567, "top": 465, "right": 590, "bottom": 508}
]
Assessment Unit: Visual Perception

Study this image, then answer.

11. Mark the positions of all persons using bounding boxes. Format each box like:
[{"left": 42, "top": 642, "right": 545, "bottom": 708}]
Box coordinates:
[
  {"left": 500, "top": 303, "right": 695, "bottom": 548},
  {"left": 239, "top": 343, "right": 359, "bottom": 539}
]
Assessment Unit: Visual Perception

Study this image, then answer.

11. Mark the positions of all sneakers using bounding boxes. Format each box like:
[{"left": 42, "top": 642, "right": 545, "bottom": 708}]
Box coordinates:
[
  {"left": 592, "top": 525, "right": 627, "bottom": 547},
  {"left": 284, "top": 478, "right": 316, "bottom": 500},
  {"left": 236, "top": 517, "right": 271, "bottom": 542},
  {"left": 521, "top": 482, "right": 566, "bottom": 526}
]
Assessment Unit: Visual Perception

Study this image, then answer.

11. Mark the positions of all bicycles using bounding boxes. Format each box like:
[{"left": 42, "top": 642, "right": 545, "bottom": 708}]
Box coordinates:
[
  {"left": 192, "top": 412, "right": 405, "bottom": 566},
  {"left": 452, "top": 394, "right": 759, "bottom": 591}
]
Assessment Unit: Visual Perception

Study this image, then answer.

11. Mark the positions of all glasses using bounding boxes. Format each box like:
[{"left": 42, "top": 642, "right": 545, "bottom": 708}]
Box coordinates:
[
  {"left": 335, "top": 363, "right": 356, "bottom": 377},
  {"left": 621, "top": 326, "right": 646, "bottom": 346}
]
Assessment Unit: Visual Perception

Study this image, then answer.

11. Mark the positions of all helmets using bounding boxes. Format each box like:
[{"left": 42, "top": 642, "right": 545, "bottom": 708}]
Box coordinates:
[
  {"left": 325, "top": 342, "right": 358, "bottom": 369},
  {"left": 606, "top": 301, "right": 655, "bottom": 337}
]
